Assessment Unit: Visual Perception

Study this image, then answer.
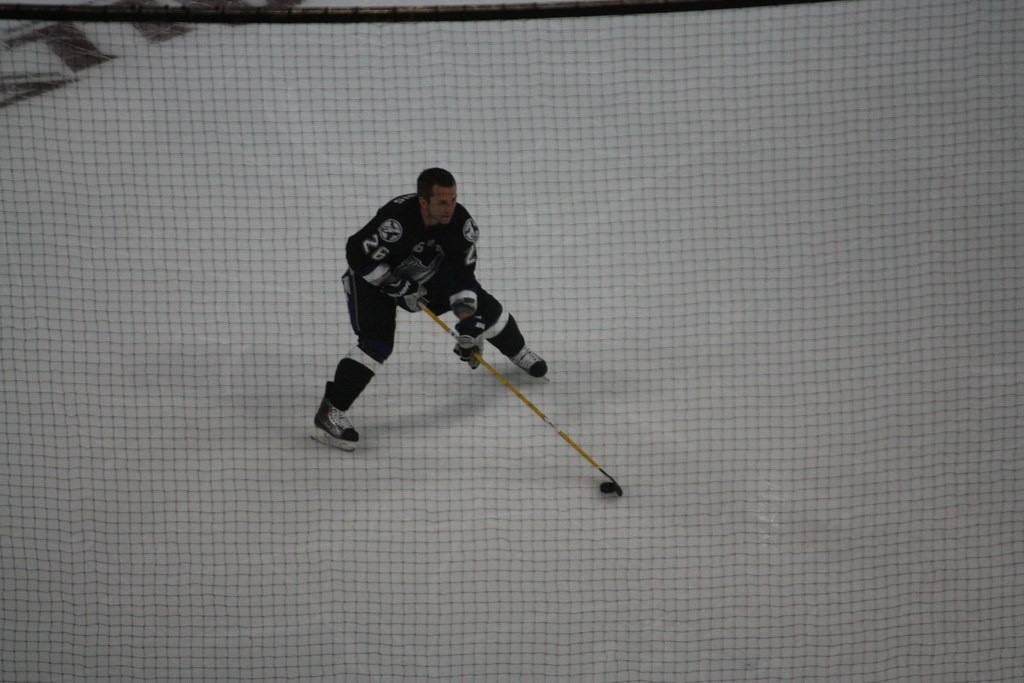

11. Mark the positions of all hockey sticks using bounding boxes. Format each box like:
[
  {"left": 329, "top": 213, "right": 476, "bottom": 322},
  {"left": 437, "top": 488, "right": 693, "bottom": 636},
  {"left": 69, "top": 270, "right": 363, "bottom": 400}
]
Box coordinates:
[{"left": 418, "top": 301, "right": 623, "bottom": 496}]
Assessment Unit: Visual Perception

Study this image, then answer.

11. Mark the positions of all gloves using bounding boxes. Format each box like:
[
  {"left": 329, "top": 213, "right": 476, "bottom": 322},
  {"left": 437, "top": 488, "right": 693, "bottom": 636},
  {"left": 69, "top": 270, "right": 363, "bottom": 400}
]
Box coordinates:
[
  {"left": 383, "top": 272, "right": 429, "bottom": 314},
  {"left": 451, "top": 314, "right": 486, "bottom": 371}
]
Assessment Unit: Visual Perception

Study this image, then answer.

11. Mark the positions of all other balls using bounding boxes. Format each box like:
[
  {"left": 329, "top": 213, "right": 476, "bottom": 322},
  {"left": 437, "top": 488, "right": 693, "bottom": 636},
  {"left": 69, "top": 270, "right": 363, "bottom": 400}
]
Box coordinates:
[{"left": 599, "top": 481, "right": 616, "bottom": 494}]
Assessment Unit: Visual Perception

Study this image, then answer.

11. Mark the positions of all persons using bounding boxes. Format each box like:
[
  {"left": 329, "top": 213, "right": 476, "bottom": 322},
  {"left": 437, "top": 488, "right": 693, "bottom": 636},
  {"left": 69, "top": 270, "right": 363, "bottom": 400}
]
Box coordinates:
[{"left": 312, "top": 166, "right": 549, "bottom": 444}]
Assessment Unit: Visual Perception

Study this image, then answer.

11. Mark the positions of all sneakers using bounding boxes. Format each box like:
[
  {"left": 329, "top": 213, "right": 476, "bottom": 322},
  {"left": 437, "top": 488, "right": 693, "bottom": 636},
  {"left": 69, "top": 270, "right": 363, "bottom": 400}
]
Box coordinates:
[
  {"left": 505, "top": 346, "right": 551, "bottom": 383},
  {"left": 307, "top": 402, "right": 360, "bottom": 453}
]
